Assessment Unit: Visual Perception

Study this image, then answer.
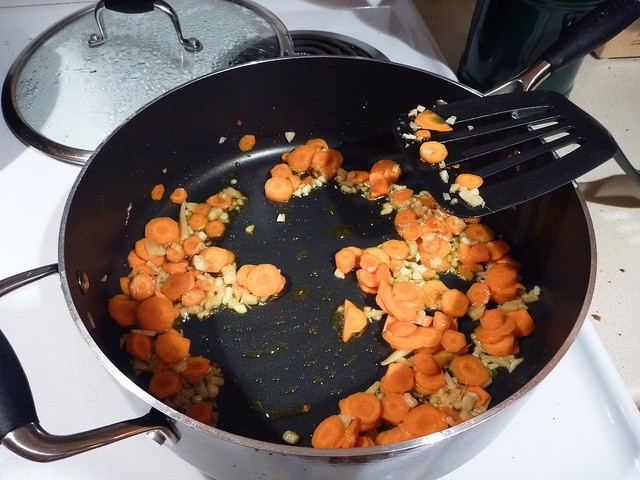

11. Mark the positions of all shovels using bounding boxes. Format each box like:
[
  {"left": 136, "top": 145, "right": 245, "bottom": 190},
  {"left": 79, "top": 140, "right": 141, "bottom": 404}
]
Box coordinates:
[{"left": 392, "top": 89, "right": 640, "bottom": 218}]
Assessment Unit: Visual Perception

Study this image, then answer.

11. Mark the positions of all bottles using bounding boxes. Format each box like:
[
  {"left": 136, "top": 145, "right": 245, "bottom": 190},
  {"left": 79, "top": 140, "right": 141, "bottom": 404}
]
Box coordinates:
[{"left": 457, "top": 0, "right": 602, "bottom": 114}]
